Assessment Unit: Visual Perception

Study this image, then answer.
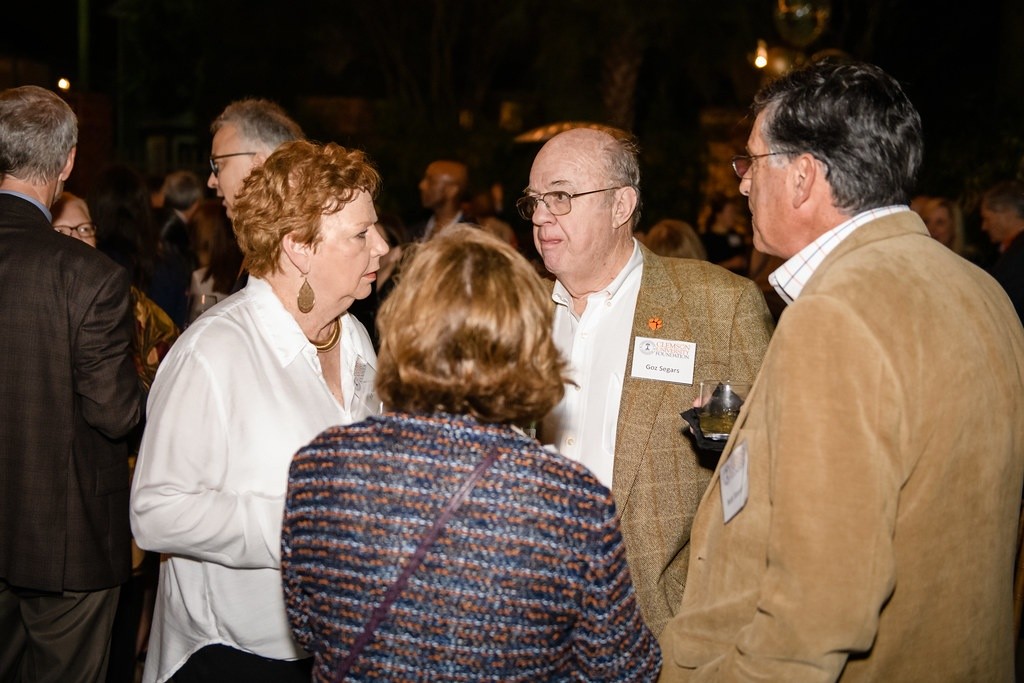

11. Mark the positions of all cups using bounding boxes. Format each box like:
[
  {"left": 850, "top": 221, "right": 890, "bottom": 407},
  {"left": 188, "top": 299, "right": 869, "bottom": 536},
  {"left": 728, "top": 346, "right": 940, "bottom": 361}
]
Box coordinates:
[
  {"left": 699, "top": 381, "right": 752, "bottom": 441},
  {"left": 184, "top": 294, "right": 217, "bottom": 331}
]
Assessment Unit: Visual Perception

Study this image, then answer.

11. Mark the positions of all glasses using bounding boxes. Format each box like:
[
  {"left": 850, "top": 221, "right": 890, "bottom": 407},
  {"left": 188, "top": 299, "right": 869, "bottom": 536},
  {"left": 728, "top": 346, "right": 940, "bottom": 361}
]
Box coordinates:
[
  {"left": 516, "top": 187, "right": 620, "bottom": 220},
  {"left": 210, "top": 152, "right": 256, "bottom": 178},
  {"left": 730, "top": 150, "right": 794, "bottom": 178},
  {"left": 53, "top": 222, "right": 97, "bottom": 238}
]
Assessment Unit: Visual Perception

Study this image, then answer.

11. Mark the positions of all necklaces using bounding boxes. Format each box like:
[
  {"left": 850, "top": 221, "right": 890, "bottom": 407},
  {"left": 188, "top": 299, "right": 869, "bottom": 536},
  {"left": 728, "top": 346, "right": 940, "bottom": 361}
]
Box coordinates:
[{"left": 305, "top": 311, "right": 342, "bottom": 352}]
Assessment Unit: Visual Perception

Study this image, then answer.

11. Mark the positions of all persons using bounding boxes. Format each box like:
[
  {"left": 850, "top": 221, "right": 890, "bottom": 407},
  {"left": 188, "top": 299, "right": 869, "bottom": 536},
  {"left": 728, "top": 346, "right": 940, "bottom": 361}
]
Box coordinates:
[
  {"left": 653, "top": 53, "right": 1024, "bottom": 681},
  {"left": 130, "top": 54, "right": 1024, "bottom": 379},
  {"left": 43, "top": 191, "right": 184, "bottom": 683},
  {"left": 121, "top": 141, "right": 388, "bottom": 683},
  {"left": 0, "top": 85, "right": 151, "bottom": 682},
  {"left": 519, "top": 124, "right": 777, "bottom": 666},
  {"left": 277, "top": 222, "right": 666, "bottom": 682}
]
